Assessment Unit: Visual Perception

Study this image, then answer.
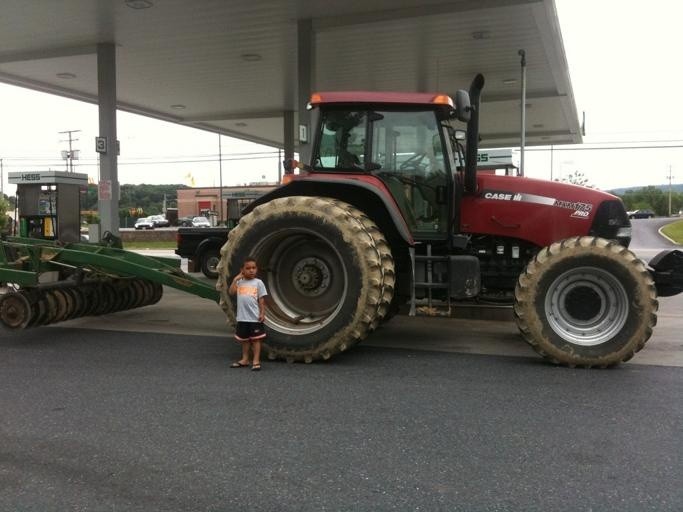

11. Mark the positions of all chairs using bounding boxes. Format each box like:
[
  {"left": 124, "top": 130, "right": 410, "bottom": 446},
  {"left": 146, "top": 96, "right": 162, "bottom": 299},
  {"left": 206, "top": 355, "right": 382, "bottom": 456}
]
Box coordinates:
[{"left": 336, "top": 126, "right": 381, "bottom": 171}]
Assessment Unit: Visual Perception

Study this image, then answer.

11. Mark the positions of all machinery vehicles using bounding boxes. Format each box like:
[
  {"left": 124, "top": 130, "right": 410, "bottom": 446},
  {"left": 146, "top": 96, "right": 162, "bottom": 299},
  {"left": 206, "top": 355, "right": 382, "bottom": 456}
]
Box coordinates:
[{"left": 215, "top": 72, "right": 683, "bottom": 370}]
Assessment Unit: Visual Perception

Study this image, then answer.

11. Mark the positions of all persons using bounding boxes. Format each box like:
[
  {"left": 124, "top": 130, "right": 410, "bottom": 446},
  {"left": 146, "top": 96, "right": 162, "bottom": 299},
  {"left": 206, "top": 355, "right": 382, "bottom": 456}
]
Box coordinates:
[{"left": 226, "top": 257, "right": 268, "bottom": 371}]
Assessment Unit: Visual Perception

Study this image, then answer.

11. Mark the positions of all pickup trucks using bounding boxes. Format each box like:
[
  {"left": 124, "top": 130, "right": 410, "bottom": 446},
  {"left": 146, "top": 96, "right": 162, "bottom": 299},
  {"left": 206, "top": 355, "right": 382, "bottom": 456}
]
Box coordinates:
[{"left": 175, "top": 226, "right": 233, "bottom": 280}]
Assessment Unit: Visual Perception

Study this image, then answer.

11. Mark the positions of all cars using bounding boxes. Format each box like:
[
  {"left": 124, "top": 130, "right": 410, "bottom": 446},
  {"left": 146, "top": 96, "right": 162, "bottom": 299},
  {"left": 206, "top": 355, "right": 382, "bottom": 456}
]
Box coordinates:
[
  {"left": 135, "top": 215, "right": 169, "bottom": 231},
  {"left": 177, "top": 216, "right": 210, "bottom": 227},
  {"left": 627, "top": 208, "right": 655, "bottom": 219}
]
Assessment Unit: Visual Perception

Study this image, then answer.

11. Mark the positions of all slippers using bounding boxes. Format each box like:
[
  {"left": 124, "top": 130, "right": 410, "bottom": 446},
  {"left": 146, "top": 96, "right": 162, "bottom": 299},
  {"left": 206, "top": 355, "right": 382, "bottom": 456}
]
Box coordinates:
[
  {"left": 249, "top": 362, "right": 261, "bottom": 372},
  {"left": 230, "top": 359, "right": 250, "bottom": 368}
]
